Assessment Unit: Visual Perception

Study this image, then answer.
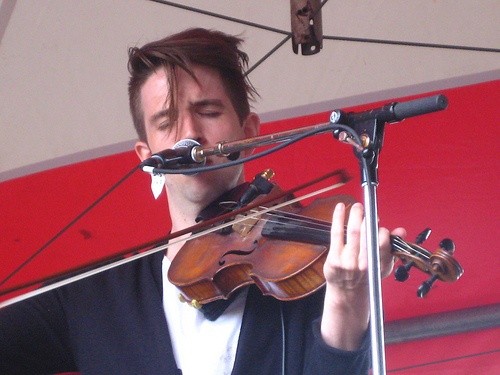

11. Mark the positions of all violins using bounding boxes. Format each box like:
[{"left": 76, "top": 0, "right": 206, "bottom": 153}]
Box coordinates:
[{"left": 167, "top": 169, "right": 464, "bottom": 310}]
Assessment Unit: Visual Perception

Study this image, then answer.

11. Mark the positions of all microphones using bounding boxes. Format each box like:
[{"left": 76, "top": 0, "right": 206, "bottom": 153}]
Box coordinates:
[{"left": 139, "top": 139, "right": 207, "bottom": 176}]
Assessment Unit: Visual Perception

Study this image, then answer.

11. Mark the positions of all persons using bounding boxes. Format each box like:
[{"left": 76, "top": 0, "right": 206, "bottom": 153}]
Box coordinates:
[{"left": 0, "top": 25, "right": 407, "bottom": 374}]
